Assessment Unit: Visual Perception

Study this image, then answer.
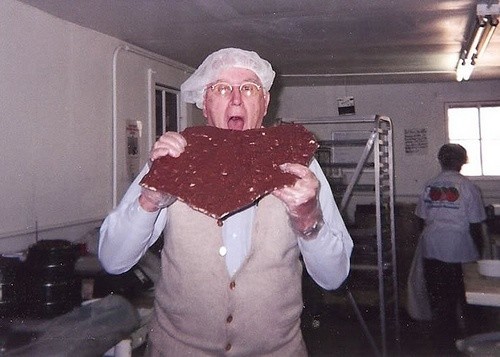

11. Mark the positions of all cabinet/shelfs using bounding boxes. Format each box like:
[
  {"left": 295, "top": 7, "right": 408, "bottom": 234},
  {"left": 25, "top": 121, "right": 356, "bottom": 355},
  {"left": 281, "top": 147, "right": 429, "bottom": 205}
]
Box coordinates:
[{"left": 279, "top": 114, "right": 400, "bottom": 357}]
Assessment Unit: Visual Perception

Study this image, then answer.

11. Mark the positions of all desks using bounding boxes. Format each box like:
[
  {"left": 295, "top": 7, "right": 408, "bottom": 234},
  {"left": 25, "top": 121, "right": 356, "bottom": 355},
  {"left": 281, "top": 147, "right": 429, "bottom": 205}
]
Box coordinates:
[{"left": 461, "top": 262, "right": 500, "bottom": 307}]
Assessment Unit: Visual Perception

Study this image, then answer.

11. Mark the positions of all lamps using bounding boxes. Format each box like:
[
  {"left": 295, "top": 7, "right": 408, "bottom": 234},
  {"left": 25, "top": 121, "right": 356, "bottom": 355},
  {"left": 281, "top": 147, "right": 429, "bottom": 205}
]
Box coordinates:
[{"left": 455, "top": 4, "right": 500, "bottom": 82}]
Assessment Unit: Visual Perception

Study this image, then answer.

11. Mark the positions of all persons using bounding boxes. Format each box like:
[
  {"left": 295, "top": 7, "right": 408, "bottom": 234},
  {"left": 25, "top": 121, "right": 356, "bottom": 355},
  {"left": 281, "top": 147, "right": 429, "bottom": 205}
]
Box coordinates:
[
  {"left": 414, "top": 143, "right": 488, "bottom": 351},
  {"left": 96, "top": 47, "right": 355, "bottom": 357}
]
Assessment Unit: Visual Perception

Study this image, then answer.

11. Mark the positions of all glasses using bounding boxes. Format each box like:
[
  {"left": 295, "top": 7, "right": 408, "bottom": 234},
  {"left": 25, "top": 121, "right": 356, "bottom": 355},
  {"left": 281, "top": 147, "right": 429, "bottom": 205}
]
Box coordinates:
[{"left": 203, "top": 81, "right": 264, "bottom": 96}]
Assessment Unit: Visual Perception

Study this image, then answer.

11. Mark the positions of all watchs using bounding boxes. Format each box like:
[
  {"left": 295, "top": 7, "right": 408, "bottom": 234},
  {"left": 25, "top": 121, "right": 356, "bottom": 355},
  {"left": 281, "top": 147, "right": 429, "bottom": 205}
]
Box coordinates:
[{"left": 288, "top": 211, "right": 325, "bottom": 238}]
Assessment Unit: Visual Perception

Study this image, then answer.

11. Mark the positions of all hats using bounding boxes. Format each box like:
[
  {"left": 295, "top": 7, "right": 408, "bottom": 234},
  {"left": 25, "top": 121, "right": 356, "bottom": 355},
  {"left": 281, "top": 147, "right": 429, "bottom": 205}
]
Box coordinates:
[{"left": 181, "top": 48, "right": 275, "bottom": 108}]
